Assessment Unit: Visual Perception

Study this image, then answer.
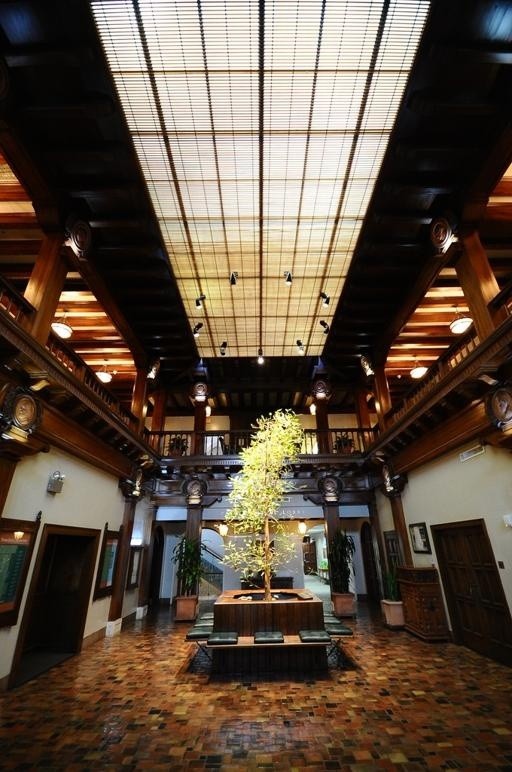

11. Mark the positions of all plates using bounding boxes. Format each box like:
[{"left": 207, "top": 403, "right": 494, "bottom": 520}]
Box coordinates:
[{"left": 396, "top": 563, "right": 454, "bottom": 644}]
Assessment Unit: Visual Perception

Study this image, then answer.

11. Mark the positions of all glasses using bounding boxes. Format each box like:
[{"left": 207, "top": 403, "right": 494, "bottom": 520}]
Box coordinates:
[
  {"left": 94, "top": 358, "right": 116, "bottom": 384},
  {"left": 407, "top": 352, "right": 429, "bottom": 382},
  {"left": 51, "top": 309, "right": 77, "bottom": 338},
  {"left": 447, "top": 301, "right": 475, "bottom": 335},
  {"left": 191, "top": 265, "right": 332, "bottom": 365}
]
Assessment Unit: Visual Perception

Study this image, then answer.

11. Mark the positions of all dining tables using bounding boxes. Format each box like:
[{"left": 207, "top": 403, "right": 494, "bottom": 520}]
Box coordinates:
[
  {"left": 166, "top": 435, "right": 188, "bottom": 456},
  {"left": 170, "top": 529, "right": 205, "bottom": 623},
  {"left": 212, "top": 407, "right": 325, "bottom": 677},
  {"left": 325, "top": 528, "right": 361, "bottom": 618},
  {"left": 373, "top": 552, "right": 409, "bottom": 627},
  {"left": 332, "top": 432, "right": 357, "bottom": 456}
]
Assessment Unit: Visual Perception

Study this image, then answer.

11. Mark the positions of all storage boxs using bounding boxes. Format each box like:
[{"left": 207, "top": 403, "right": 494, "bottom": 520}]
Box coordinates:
[
  {"left": 184, "top": 610, "right": 213, "bottom": 666},
  {"left": 324, "top": 605, "right": 354, "bottom": 661},
  {"left": 206, "top": 630, "right": 332, "bottom": 672}
]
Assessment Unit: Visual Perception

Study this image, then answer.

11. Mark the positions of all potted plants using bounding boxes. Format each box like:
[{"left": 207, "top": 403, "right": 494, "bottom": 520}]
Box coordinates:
[
  {"left": 93, "top": 521, "right": 124, "bottom": 602},
  {"left": 406, "top": 522, "right": 433, "bottom": 555},
  {"left": 0, "top": 509, "right": 44, "bottom": 627},
  {"left": 125, "top": 543, "right": 144, "bottom": 592}
]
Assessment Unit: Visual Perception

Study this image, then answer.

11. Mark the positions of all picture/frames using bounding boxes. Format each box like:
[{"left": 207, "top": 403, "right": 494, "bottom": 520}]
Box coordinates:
[
  {"left": 238, "top": 574, "right": 294, "bottom": 590},
  {"left": 317, "top": 566, "right": 329, "bottom": 584}
]
